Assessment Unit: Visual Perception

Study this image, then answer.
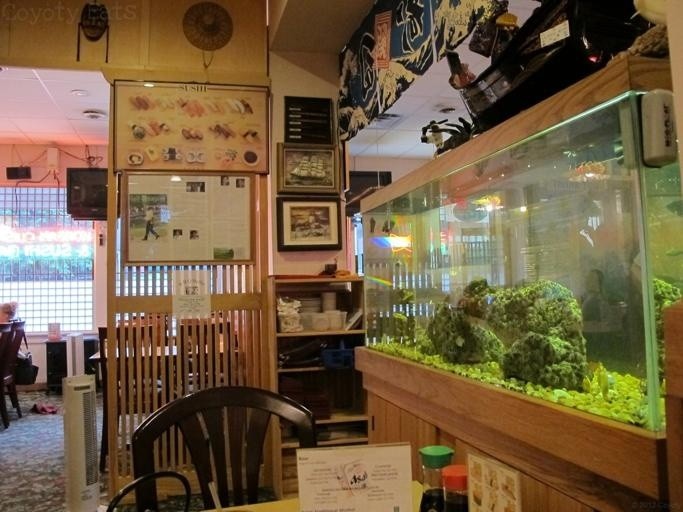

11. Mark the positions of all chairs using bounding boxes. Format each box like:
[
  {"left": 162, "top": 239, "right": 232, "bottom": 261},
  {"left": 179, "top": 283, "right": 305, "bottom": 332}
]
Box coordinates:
[
  {"left": 131, "top": 387, "right": 318, "bottom": 512},
  {"left": 88, "top": 311, "right": 245, "bottom": 474},
  {"left": 0, "top": 321, "right": 26, "bottom": 430}
]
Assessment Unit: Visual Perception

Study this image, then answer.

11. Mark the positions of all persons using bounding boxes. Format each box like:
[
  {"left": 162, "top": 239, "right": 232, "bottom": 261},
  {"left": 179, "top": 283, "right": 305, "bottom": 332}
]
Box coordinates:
[
  {"left": 235, "top": 179, "right": 244, "bottom": 188},
  {"left": 141, "top": 206, "right": 159, "bottom": 240},
  {"left": 173, "top": 229, "right": 181, "bottom": 240},
  {"left": 190, "top": 230, "right": 199, "bottom": 240},
  {"left": 220, "top": 176, "right": 230, "bottom": 186}
]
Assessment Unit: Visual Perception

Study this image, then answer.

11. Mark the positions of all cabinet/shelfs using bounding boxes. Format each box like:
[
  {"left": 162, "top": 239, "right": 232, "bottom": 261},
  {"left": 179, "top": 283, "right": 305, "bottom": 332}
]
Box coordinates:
[
  {"left": 44, "top": 335, "right": 100, "bottom": 396},
  {"left": 265, "top": 273, "right": 369, "bottom": 501}
]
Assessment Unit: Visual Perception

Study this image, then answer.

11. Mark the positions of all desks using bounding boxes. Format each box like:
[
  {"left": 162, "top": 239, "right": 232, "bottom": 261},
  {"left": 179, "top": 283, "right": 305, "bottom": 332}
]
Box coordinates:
[{"left": 198, "top": 480, "right": 429, "bottom": 512}]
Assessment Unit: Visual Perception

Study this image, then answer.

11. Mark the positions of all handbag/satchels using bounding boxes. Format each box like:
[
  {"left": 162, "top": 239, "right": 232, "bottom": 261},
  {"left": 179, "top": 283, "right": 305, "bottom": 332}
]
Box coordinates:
[
  {"left": 15, "top": 366, "right": 38, "bottom": 386},
  {"left": 16, "top": 333, "right": 32, "bottom": 367}
]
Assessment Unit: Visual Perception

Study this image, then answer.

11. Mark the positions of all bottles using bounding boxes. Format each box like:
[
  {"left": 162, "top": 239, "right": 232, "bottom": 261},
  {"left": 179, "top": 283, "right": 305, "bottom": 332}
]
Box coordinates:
[
  {"left": 418, "top": 444, "right": 470, "bottom": 512},
  {"left": 445, "top": 51, "right": 497, "bottom": 110}
]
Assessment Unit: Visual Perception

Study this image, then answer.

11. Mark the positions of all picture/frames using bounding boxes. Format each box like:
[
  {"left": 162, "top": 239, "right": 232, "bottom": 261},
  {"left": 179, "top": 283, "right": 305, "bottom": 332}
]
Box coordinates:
[
  {"left": 110, "top": 88, "right": 269, "bottom": 267},
  {"left": 275, "top": 140, "right": 345, "bottom": 253}
]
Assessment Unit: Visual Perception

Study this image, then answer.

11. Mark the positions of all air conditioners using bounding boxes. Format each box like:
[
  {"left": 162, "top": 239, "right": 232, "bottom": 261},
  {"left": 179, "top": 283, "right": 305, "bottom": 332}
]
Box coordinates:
[
  {"left": 66, "top": 332, "right": 85, "bottom": 377},
  {"left": 61, "top": 373, "right": 101, "bottom": 512}
]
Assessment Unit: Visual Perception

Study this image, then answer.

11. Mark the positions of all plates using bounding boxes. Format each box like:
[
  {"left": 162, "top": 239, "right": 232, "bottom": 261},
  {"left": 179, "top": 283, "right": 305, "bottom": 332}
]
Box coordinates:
[{"left": 294, "top": 296, "right": 320, "bottom": 312}]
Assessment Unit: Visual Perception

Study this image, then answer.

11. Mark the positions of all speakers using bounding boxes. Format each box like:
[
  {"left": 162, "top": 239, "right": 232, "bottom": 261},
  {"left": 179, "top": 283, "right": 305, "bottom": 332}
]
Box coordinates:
[{"left": 6, "top": 166, "right": 32, "bottom": 179}]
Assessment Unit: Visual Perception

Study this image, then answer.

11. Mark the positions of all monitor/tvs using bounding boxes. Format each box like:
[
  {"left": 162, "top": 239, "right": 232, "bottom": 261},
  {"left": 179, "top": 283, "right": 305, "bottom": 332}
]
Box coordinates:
[{"left": 66, "top": 168, "right": 121, "bottom": 220}]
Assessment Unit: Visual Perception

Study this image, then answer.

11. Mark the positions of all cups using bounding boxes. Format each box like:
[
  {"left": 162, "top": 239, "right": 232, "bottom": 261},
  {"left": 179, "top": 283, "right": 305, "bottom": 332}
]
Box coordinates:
[{"left": 312, "top": 292, "right": 347, "bottom": 331}]
